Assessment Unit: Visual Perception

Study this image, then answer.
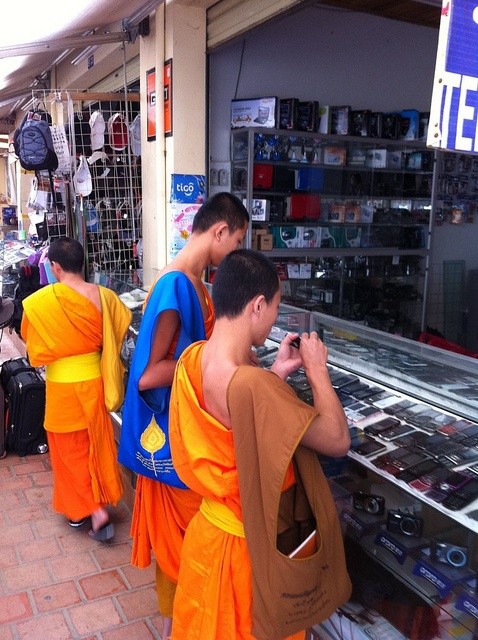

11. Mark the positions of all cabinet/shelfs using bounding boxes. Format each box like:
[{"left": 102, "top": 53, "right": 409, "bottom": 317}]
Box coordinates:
[
  {"left": 230, "top": 127, "right": 442, "bottom": 340},
  {"left": 115, "top": 279, "right": 478, "bottom": 639}
]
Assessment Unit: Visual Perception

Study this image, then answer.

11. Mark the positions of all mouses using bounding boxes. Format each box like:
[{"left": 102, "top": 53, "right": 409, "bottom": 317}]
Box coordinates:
[
  {"left": 303, "top": 230, "right": 315, "bottom": 240},
  {"left": 282, "top": 230, "right": 293, "bottom": 240}
]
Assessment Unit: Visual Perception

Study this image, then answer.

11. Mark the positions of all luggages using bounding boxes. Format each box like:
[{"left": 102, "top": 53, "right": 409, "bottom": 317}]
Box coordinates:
[{"left": 0, "top": 357, "right": 49, "bottom": 457}]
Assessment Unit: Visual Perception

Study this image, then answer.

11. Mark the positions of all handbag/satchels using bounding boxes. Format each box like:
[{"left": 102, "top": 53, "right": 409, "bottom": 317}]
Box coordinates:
[
  {"left": 226, "top": 365, "right": 353, "bottom": 640},
  {"left": 117, "top": 272, "right": 206, "bottom": 490},
  {"left": 97, "top": 285, "right": 133, "bottom": 412}
]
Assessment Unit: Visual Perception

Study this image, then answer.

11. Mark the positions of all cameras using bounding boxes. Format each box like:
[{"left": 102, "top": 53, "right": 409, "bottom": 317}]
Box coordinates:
[
  {"left": 429, "top": 536, "right": 470, "bottom": 572},
  {"left": 386, "top": 508, "right": 424, "bottom": 540},
  {"left": 353, "top": 493, "right": 385, "bottom": 519}
]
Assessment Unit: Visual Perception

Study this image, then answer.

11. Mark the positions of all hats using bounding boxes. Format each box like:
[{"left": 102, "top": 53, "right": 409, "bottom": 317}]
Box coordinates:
[
  {"left": 89, "top": 110, "right": 105, "bottom": 151},
  {"left": 73, "top": 155, "right": 92, "bottom": 197},
  {"left": 129, "top": 114, "right": 141, "bottom": 154},
  {"left": 108, "top": 113, "right": 128, "bottom": 151}
]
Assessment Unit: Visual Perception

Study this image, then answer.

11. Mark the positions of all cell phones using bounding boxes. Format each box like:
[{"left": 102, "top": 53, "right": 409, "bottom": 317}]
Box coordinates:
[
  {"left": 254, "top": 347, "right": 375, "bottom": 458},
  {"left": 375, "top": 399, "right": 477, "bottom": 512},
  {"left": 284, "top": 331, "right": 301, "bottom": 349},
  {"left": 322, "top": 330, "right": 478, "bottom": 401}
]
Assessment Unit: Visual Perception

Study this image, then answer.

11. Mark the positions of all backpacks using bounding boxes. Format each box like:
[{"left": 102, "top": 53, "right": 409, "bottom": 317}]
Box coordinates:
[{"left": 13, "top": 108, "right": 58, "bottom": 172}]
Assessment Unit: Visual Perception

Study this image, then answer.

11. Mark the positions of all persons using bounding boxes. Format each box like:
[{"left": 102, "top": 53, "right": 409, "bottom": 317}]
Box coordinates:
[
  {"left": 19, "top": 236, "right": 134, "bottom": 543},
  {"left": 168, "top": 247, "right": 354, "bottom": 640},
  {"left": 116, "top": 191, "right": 251, "bottom": 634}
]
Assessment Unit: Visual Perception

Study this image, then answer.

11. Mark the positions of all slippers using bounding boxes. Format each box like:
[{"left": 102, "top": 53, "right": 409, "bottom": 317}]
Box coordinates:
[
  {"left": 88, "top": 522, "right": 115, "bottom": 542},
  {"left": 68, "top": 518, "right": 87, "bottom": 527}
]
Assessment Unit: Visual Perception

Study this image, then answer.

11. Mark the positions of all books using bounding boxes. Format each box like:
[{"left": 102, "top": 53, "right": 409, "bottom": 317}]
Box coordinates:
[{"left": 287, "top": 526, "right": 317, "bottom": 560}]
